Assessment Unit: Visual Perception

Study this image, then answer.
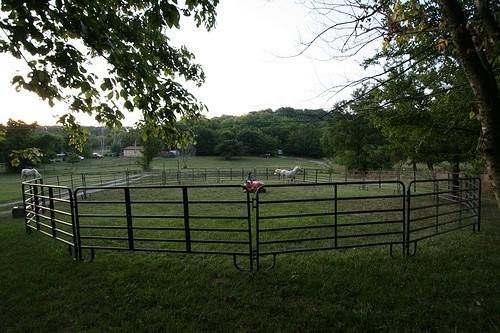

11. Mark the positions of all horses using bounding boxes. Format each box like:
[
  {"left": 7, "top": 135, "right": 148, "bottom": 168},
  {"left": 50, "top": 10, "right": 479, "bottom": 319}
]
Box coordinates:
[
  {"left": 280, "top": 166, "right": 303, "bottom": 182},
  {"left": 21, "top": 169, "right": 41, "bottom": 181},
  {"left": 274, "top": 169, "right": 282, "bottom": 180}
]
